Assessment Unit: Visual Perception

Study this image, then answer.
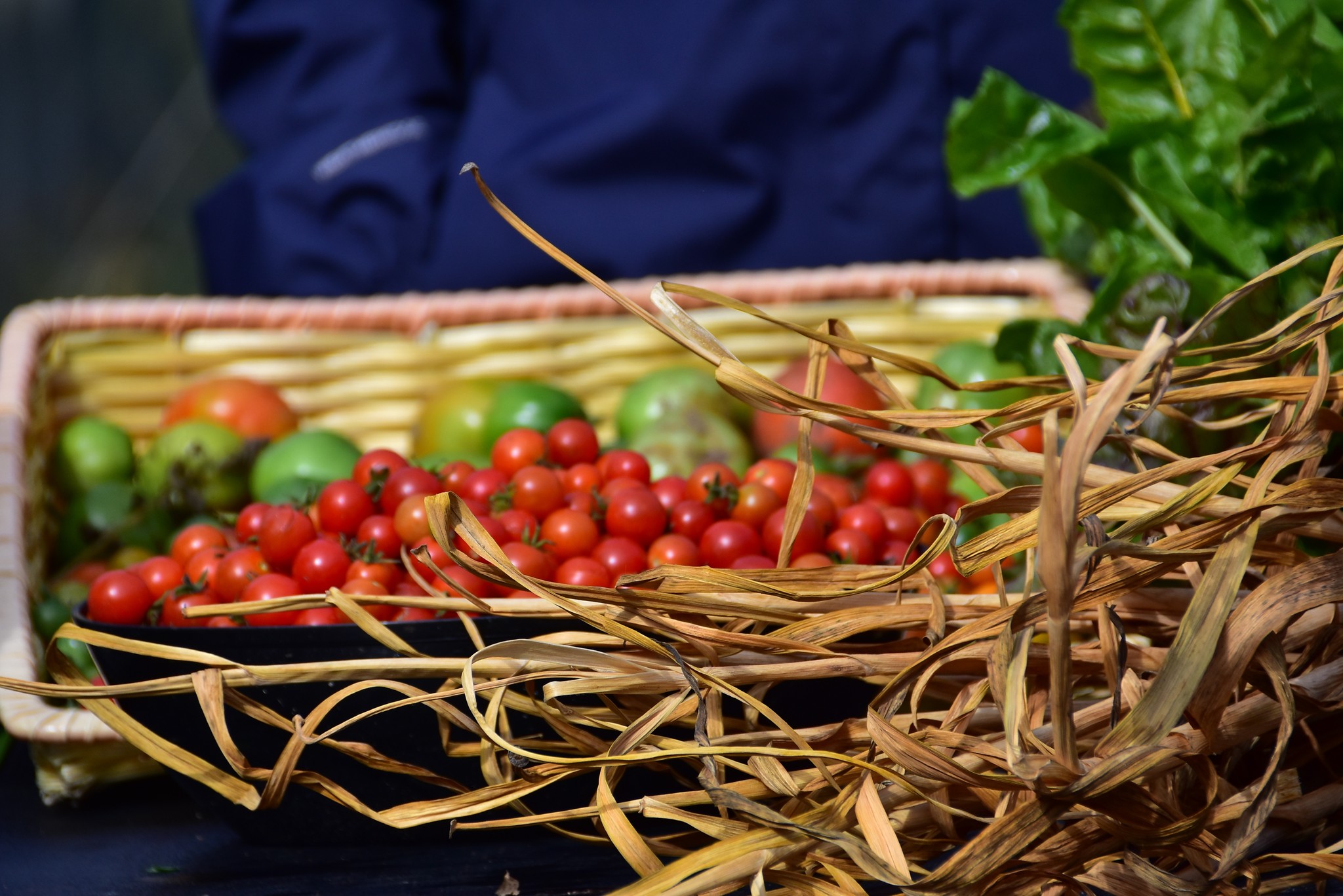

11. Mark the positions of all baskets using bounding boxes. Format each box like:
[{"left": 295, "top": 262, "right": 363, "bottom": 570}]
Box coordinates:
[{"left": 2, "top": 259, "right": 1095, "bottom": 790}]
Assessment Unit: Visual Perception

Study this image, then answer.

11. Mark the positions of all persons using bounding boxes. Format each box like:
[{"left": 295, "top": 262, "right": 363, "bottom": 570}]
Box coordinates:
[{"left": 192, "top": 0, "right": 1096, "bottom": 295}]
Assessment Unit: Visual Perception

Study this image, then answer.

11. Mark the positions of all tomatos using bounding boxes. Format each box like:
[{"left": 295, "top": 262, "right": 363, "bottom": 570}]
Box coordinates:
[{"left": 35, "top": 350, "right": 1056, "bottom": 697}]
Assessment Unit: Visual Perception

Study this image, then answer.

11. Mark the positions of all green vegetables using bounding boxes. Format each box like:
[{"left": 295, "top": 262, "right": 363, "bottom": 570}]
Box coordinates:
[{"left": 943, "top": 0, "right": 1343, "bottom": 480}]
